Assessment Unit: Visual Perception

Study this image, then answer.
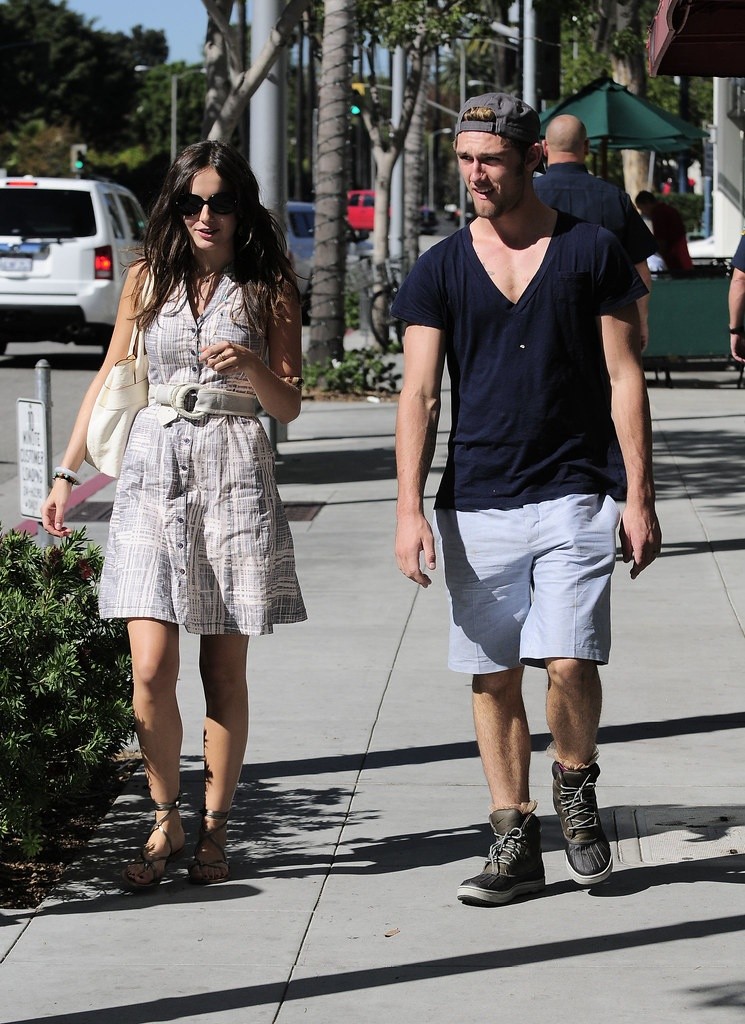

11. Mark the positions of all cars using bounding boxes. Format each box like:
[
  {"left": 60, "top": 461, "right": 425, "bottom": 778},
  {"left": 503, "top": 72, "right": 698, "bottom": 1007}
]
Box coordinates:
[
  {"left": 284, "top": 201, "right": 358, "bottom": 265},
  {"left": 344, "top": 189, "right": 393, "bottom": 235},
  {"left": 1, "top": 177, "right": 157, "bottom": 347}
]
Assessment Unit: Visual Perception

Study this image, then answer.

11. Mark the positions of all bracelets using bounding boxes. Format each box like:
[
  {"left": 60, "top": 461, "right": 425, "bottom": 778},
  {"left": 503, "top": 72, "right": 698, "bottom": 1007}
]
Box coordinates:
[
  {"left": 729, "top": 326, "right": 743, "bottom": 334},
  {"left": 52, "top": 467, "right": 82, "bottom": 486}
]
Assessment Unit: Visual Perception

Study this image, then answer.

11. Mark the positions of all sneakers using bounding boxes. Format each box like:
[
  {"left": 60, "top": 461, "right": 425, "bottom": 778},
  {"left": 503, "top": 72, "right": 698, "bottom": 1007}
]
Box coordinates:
[
  {"left": 457, "top": 808, "right": 546, "bottom": 905},
  {"left": 552, "top": 763, "right": 613, "bottom": 884}
]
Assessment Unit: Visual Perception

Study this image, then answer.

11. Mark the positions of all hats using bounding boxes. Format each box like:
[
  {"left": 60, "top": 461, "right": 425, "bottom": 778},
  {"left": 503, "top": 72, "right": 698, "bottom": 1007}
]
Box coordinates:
[{"left": 453, "top": 93, "right": 547, "bottom": 175}]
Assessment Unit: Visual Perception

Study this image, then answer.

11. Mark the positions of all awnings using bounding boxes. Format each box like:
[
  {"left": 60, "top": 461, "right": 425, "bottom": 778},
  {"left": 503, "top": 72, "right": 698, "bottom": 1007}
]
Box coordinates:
[{"left": 645, "top": 0, "right": 745, "bottom": 78}]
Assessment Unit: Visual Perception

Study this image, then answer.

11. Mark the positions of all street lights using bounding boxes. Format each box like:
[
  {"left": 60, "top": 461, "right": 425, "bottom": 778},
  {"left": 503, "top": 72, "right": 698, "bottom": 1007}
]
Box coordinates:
[
  {"left": 427, "top": 128, "right": 452, "bottom": 212},
  {"left": 134, "top": 64, "right": 214, "bottom": 168}
]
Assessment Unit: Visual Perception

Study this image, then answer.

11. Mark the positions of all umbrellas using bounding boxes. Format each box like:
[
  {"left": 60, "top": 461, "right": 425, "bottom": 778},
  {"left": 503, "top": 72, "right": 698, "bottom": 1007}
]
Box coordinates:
[{"left": 539, "top": 76, "right": 710, "bottom": 182}]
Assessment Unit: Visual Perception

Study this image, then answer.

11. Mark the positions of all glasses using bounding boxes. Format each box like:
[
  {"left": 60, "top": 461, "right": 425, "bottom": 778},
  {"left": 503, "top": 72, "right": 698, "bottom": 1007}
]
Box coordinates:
[{"left": 175, "top": 191, "right": 237, "bottom": 216}]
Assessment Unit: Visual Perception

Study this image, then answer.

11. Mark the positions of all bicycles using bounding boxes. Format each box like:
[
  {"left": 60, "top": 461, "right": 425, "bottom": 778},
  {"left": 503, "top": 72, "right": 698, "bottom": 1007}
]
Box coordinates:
[{"left": 367, "top": 256, "right": 407, "bottom": 351}]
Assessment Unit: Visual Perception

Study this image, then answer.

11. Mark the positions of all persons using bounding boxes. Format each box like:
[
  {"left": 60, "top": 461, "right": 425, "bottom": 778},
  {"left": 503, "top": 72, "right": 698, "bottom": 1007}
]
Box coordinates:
[
  {"left": 635, "top": 190, "right": 693, "bottom": 277},
  {"left": 533, "top": 115, "right": 658, "bottom": 502},
  {"left": 728, "top": 234, "right": 745, "bottom": 361},
  {"left": 41, "top": 139, "right": 301, "bottom": 883},
  {"left": 391, "top": 93, "right": 662, "bottom": 903}
]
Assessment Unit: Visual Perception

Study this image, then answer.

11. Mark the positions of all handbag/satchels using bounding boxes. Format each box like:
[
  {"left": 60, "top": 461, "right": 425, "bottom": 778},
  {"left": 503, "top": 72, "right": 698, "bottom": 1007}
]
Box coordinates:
[{"left": 85, "top": 257, "right": 149, "bottom": 480}]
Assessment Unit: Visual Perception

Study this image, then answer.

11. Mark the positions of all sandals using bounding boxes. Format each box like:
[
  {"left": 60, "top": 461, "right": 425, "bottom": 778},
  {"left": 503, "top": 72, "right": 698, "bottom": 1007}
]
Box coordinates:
[
  {"left": 121, "top": 791, "right": 185, "bottom": 889},
  {"left": 190, "top": 808, "right": 231, "bottom": 883}
]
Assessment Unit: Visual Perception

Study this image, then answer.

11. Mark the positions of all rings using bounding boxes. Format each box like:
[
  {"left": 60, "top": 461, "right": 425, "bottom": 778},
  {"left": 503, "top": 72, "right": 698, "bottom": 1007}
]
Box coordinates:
[{"left": 219, "top": 353, "right": 226, "bottom": 361}]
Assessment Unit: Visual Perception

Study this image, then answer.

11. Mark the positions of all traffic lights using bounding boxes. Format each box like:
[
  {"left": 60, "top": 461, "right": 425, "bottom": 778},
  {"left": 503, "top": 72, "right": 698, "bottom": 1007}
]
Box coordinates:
[
  {"left": 352, "top": 89, "right": 365, "bottom": 117},
  {"left": 75, "top": 149, "right": 84, "bottom": 171}
]
